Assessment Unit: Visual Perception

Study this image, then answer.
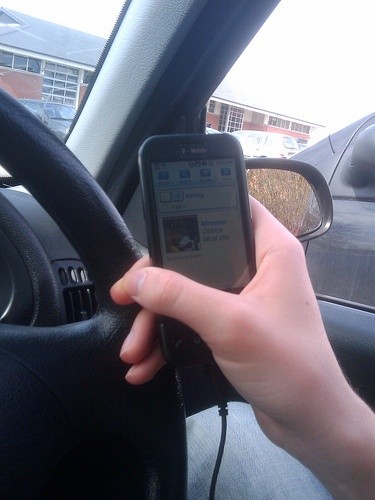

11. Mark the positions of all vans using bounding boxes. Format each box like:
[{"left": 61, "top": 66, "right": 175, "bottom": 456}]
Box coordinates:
[{"left": 231, "top": 129, "right": 298, "bottom": 159}]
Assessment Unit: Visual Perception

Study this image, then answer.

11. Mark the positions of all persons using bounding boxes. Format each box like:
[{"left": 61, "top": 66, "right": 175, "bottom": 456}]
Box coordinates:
[{"left": 109, "top": 194, "right": 375, "bottom": 500}]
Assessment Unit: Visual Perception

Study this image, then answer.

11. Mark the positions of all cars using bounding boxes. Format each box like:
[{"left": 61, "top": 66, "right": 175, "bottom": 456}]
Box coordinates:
[
  {"left": 17, "top": 98, "right": 77, "bottom": 142},
  {"left": 285, "top": 111, "right": 375, "bottom": 308}
]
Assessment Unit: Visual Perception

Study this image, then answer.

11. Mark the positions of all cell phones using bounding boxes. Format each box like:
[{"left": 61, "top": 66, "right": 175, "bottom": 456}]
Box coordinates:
[{"left": 138, "top": 133, "right": 257, "bottom": 374}]
